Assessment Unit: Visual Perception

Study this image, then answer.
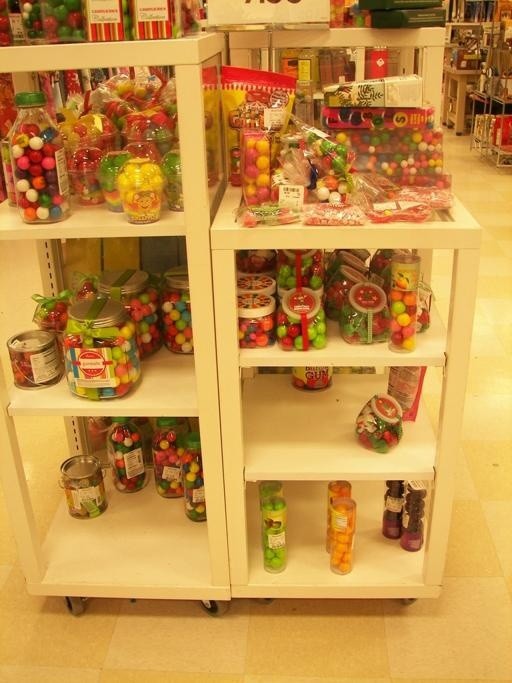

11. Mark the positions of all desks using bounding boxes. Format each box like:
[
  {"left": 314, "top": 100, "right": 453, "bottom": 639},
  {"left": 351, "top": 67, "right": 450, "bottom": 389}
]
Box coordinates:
[{"left": 441, "top": 68, "right": 482, "bottom": 137}]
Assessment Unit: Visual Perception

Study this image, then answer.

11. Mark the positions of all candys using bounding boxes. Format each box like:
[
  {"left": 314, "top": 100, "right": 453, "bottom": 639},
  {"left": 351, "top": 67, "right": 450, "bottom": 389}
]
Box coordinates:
[
  {"left": 62, "top": 415, "right": 208, "bottom": 518},
  {"left": 237, "top": 248, "right": 433, "bottom": 352},
  {"left": 384, "top": 480, "right": 427, "bottom": 553},
  {"left": 2, "top": 0, "right": 199, "bottom": 46},
  {"left": 1, "top": 73, "right": 185, "bottom": 224},
  {"left": 261, "top": 480, "right": 288, "bottom": 572},
  {"left": 354, "top": 393, "right": 405, "bottom": 455},
  {"left": 6, "top": 261, "right": 194, "bottom": 400},
  {"left": 226, "top": 98, "right": 456, "bottom": 225},
  {"left": 326, "top": 480, "right": 358, "bottom": 575},
  {"left": 289, "top": 366, "right": 333, "bottom": 390}
]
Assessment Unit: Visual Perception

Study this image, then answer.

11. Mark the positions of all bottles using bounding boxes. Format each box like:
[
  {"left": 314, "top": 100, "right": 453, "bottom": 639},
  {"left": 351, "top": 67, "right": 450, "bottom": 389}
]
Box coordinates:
[
  {"left": 259, "top": 481, "right": 425, "bottom": 576},
  {"left": 59, "top": 416, "right": 210, "bottom": 524},
  {"left": 207, "top": 93, "right": 427, "bottom": 229},
  {"left": 234, "top": 248, "right": 427, "bottom": 352},
  {"left": 480, "top": 69, "right": 487, "bottom": 94},
  {"left": 287, "top": 366, "right": 427, "bottom": 454},
  {"left": 8, "top": 91, "right": 184, "bottom": 226},
  {"left": 9, "top": 262, "right": 194, "bottom": 404}
]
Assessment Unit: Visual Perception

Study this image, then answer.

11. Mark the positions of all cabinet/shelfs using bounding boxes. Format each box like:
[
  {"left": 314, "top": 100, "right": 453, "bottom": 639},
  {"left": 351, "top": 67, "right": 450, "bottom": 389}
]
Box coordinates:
[
  {"left": 269, "top": 26, "right": 445, "bottom": 135},
  {"left": 210, "top": 180, "right": 484, "bottom": 599},
  {"left": 0, "top": 32, "right": 232, "bottom": 601},
  {"left": 469, "top": 91, "right": 512, "bottom": 169}
]
